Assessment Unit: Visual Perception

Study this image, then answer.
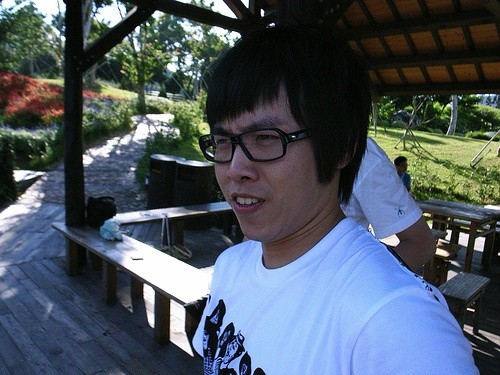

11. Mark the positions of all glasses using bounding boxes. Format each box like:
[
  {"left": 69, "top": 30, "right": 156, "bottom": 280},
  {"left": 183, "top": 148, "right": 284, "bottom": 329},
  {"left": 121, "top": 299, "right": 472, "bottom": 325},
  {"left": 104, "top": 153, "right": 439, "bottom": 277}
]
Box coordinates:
[{"left": 198, "top": 129, "right": 310, "bottom": 164}]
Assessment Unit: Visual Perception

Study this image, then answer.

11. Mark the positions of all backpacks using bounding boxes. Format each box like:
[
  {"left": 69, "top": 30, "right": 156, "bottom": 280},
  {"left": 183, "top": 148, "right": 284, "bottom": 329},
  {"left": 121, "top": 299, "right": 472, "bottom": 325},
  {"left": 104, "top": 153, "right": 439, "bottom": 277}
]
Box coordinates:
[{"left": 86, "top": 195, "right": 117, "bottom": 229}]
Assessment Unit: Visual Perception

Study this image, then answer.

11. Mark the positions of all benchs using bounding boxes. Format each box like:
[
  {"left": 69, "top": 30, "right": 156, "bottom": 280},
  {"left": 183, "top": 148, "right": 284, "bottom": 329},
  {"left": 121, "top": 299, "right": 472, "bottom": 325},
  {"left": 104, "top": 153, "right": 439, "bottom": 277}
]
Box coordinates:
[
  {"left": 51, "top": 202, "right": 232, "bottom": 344},
  {"left": 421, "top": 214, "right": 500, "bottom": 258},
  {"left": 435, "top": 272, "right": 491, "bottom": 334}
]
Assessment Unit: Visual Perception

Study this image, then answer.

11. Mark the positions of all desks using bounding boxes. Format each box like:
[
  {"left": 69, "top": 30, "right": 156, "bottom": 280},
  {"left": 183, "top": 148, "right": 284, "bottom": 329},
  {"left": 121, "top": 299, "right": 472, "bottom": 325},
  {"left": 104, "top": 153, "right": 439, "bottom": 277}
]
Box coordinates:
[{"left": 415, "top": 199, "right": 500, "bottom": 272}]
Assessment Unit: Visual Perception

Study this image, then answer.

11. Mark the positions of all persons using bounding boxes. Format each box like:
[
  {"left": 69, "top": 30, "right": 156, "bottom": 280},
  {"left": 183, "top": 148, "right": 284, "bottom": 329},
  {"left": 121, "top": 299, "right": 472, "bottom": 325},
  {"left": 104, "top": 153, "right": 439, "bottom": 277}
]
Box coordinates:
[
  {"left": 191, "top": 22, "right": 482, "bottom": 375},
  {"left": 337, "top": 136, "right": 437, "bottom": 274},
  {"left": 394, "top": 155, "right": 411, "bottom": 193}
]
